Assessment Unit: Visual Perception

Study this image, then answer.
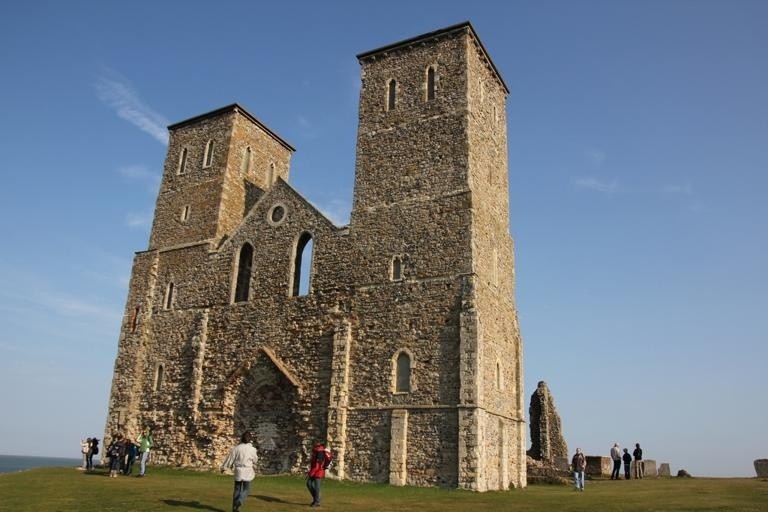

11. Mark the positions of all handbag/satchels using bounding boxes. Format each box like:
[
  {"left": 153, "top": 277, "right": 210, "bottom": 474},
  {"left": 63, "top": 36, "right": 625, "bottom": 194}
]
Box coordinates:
[{"left": 324, "top": 450, "right": 333, "bottom": 468}]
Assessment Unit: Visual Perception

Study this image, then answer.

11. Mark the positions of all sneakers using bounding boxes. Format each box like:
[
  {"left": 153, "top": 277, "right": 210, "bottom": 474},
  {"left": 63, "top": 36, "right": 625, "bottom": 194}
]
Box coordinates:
[
  {"left": 232, "top": 500, "right": 243, "bottom": 512},
  {"left": 311, "top": 501, "right": 320, "bottom": 507},
  {"left": 110, "top": 469, "right": 145, "bottom": 477}
]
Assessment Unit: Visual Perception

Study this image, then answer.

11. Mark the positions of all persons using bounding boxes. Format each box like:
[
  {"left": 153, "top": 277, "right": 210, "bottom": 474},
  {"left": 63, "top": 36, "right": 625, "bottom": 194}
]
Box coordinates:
[
  {"left": 306, "top": 437, "right": 326, "bottom": 507},
  {"left": 221, "top": 429, "right": 258, "bottom": 512},
  {"left": 632, "top": 442, "right": 645, "bottom": 480},
  {"left": 572, "top": 447, "right": 587, "bottom": 491},
  {"left": 622, "top": 447, "right": 632, "bottom": 479},
  {"left": 79, "top": 425, "right": 154, "bottom": 477},
  {"left": 610, "top": 443, "right": 622, "bottom": 480}
]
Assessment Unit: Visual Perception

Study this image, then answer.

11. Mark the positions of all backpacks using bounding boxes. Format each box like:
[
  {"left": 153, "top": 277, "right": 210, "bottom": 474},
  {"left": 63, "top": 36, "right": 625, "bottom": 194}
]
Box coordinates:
[{"left": 108, "top": 441, "right": 121, "bottom": 457}]
